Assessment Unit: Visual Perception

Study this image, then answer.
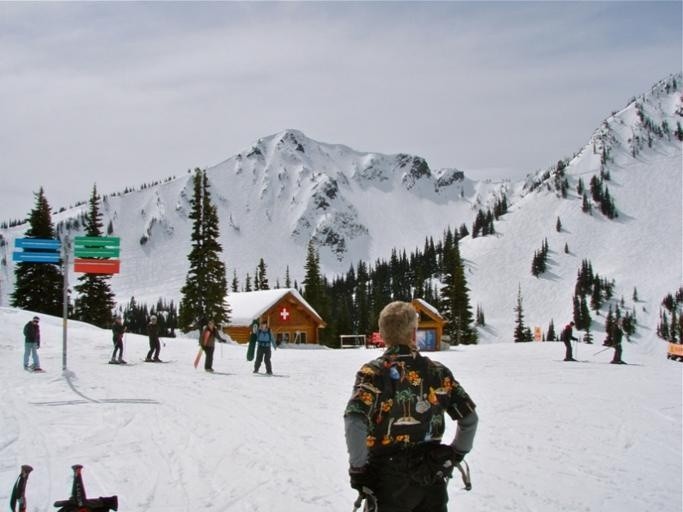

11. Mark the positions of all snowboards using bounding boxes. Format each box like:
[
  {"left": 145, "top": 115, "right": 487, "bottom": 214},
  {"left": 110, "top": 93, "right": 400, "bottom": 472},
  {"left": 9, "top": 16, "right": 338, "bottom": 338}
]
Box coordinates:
[
  {"left": 246, "top": 319, "right": 259, "bottom": 361},
  {"left": 194, "top": 347, "right": 204, "bottom": 368}
]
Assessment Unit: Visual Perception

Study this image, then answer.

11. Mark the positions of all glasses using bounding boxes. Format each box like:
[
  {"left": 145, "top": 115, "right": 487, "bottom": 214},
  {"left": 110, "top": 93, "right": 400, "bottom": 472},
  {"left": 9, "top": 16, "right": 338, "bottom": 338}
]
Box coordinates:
[{"left": 34, "top": 320, "right": 38, "bottom": 323}]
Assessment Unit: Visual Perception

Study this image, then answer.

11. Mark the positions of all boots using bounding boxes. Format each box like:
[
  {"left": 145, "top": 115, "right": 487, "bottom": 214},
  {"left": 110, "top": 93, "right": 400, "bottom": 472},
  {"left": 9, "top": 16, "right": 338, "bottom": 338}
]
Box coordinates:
[
  {"left": 109, "top": 360, "right": 127, "bottom": 364},
  {"left": 146, "top": 358, "right": 161, "bottom": 362}
]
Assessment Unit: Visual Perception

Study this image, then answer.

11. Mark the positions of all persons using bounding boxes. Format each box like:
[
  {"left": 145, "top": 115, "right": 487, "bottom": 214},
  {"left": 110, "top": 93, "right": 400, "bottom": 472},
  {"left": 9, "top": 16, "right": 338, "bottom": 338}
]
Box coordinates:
[
  {"left": 344, "top": 301, "right": 478, "bottom": 512},
  {"left": 199, "top": 320, "right": 226, "bottom": 372},
  {"left": 109, "top": 317, "right": 128, "bottom": 364},
  {"left": 23, "top": 316, "right": 42, "bottom": 371},
  {"left": 146, "top": 315, "right": 161, "bottom": 362},
  {"left": 252, "top": 320, "right": 276, "bottom": 375},
  {"left": 611, "top": 318, "right": 627, "bottom": 363},
  {"left": 561, "top": 322, "right": 578, "bottom": 361}
]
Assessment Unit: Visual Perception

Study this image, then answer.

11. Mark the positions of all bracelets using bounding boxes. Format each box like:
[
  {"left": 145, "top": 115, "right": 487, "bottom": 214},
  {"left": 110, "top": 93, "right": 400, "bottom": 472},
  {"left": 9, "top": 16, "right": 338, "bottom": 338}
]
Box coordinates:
[{"left": 349, "top": 463, "right": 370, "bottom": 472}]
